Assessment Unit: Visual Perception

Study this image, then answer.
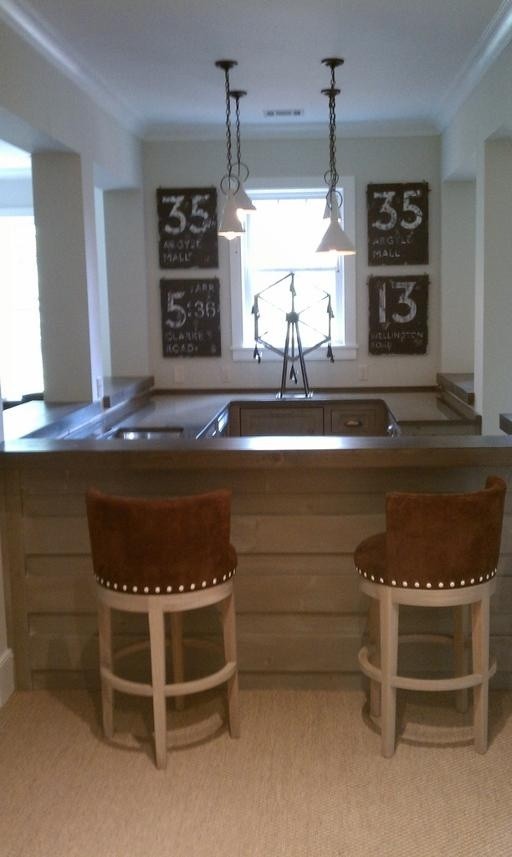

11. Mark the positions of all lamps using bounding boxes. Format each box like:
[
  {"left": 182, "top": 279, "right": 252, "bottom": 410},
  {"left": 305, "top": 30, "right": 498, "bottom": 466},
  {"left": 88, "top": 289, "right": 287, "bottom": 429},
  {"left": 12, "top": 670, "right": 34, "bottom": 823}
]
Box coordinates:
[
  {"left": 215, "top": 58, "right": 247, "bottom": 241},
  {"left": 215, "top": 85, "right": 257, "bottom": 211},
  {"left": 320, "top": 87, "right": 344, "bottom": 220},
  {"left": 314, "top": 56, "right": 358, "bottom": 257}
]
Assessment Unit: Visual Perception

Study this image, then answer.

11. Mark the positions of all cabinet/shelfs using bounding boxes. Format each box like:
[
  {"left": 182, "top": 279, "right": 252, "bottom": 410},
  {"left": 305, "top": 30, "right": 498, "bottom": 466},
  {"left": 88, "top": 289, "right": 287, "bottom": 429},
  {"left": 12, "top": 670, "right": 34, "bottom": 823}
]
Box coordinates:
[{"left": 231, "top": 397, "right": 390, "bottom": 436}]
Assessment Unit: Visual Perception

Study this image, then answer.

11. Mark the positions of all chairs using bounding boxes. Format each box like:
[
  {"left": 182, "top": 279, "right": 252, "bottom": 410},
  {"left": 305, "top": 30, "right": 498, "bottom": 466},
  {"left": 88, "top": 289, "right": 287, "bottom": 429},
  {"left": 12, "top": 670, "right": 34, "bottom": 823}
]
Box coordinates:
[
  {"left": 351, "top": 475, "right": 508, "bottom": 759},
  {"left": 86, "top": 485, "right": 246, "bottom": 772}
]
